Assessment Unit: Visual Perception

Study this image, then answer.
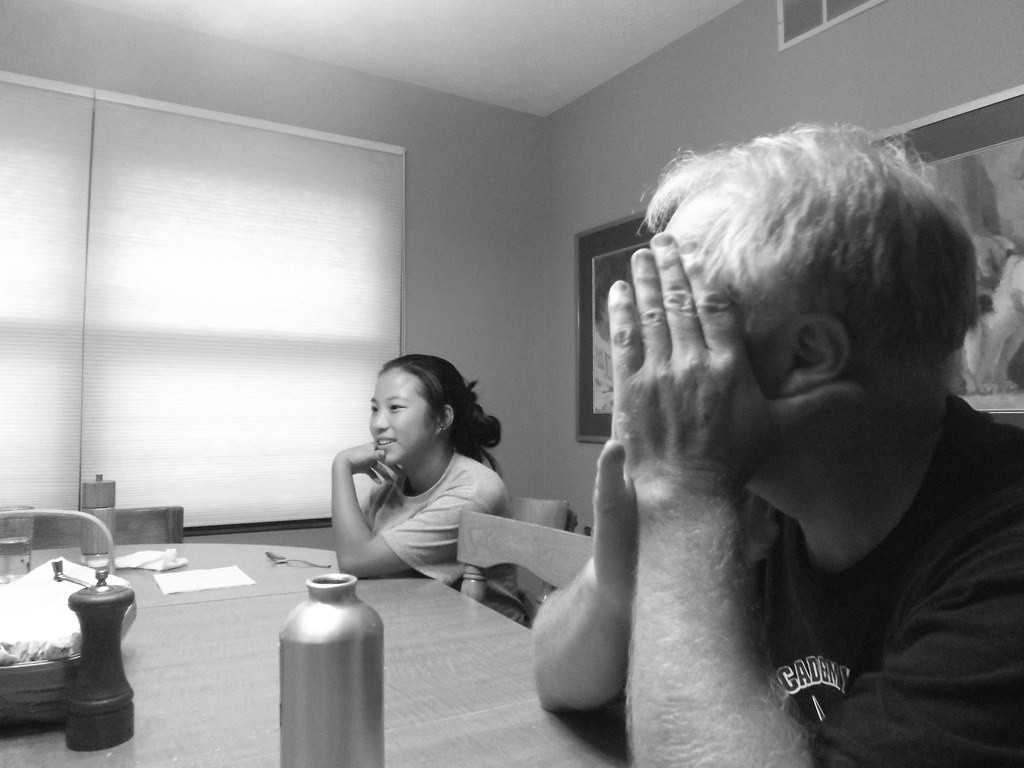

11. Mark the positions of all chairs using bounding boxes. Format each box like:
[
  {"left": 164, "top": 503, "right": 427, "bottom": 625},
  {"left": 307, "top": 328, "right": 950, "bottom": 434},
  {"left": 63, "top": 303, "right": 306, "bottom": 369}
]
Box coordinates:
[
  {"left": 29, "top": 506, "right": 188, "bottom": 557},
  {"left": 455, "top": 506, "right": 601, "bottom": 641},
  {"left": 503, "top": 495, "right": 576, "bottom": 613}
]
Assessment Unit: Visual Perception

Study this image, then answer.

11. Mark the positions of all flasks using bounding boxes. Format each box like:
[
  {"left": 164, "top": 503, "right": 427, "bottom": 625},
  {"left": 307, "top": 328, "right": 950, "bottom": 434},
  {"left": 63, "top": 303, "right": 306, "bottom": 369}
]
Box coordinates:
[{"left": 81, "top": 474, "right": 115, "bottom": 570}]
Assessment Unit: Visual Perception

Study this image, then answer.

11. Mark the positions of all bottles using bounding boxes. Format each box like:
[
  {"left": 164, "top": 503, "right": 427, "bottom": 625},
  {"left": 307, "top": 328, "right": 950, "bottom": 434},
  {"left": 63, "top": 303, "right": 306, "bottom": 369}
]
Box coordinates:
[{"left": 277, "top": 573, "right": 388, "bottom": 768}]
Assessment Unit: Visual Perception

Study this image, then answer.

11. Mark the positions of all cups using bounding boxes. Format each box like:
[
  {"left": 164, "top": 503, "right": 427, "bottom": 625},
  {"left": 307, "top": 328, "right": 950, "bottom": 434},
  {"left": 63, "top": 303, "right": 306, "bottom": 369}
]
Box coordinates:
[{"left": 0, "top": 506, "right": 34, "bottom": 583}]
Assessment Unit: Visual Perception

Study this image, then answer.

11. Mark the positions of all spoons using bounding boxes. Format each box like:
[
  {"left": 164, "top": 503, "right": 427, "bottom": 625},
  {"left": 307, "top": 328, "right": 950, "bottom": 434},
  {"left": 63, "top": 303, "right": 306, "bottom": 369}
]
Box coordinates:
[{"left": 264, "top": 551, "right": 332, "bottom": 568}]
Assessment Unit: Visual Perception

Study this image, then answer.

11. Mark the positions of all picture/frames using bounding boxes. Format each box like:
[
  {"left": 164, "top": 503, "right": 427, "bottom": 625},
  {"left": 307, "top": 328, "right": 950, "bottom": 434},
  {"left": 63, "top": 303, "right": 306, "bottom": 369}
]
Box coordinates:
[
  {"left": 572, "top": 209, "right": 669, "bottom": 448},
  {"left": 870, "top": 89, "right": 1024, "bottom": 420}
]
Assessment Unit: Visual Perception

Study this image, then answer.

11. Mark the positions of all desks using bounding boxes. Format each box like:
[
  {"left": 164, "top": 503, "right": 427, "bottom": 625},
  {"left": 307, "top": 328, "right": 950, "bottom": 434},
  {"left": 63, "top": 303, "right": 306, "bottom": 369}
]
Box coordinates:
[{"left": 0, "top": 540, "right": 633, "bottom": 767}]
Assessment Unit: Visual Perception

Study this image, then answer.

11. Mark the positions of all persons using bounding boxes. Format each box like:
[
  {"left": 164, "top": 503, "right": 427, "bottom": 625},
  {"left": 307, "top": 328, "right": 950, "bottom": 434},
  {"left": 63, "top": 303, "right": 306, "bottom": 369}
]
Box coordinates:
[
  {"left": 329, "top": 354, "right": 528, "bottom": 627},
  {"left": 528, "top": 123, "right": 1023, "bottom": 767}
]
